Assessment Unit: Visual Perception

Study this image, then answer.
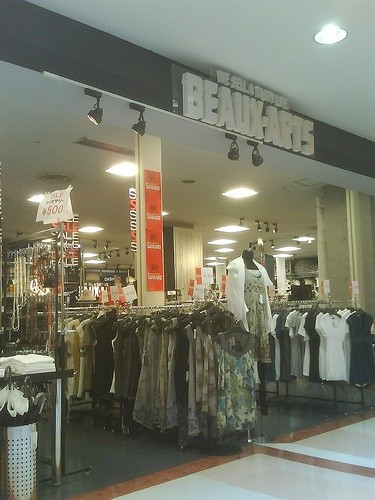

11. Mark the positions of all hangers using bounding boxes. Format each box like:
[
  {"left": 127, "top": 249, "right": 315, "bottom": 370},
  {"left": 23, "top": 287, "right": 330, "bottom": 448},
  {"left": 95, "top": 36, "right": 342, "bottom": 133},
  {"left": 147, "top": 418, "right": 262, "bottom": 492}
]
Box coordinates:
[
  {"left": 70, "top": 296, "right": 260, "bottom": 342},
  {"left": 270, "top": 299, "right": 359, "bottom": 314}
]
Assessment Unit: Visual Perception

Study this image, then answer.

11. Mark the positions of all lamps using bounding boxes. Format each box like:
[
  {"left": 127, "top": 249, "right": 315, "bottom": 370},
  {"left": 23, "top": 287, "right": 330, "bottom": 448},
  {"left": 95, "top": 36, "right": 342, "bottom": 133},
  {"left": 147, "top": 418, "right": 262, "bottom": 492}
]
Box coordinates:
[
  {"left": 264, "top": 222, "right": 271, "bottom": 232},
  {"left": 273, "top": 223, "right": 278, "bottom": 234},
  {"left": 256, "top": 221, "right": 262, "bottom": 232},
  {"left": 84, "top": 89, "right": 103, "bottom": 125},
  {"left": 129, "top": 103, "right": 146, "bottom": 137},
  {"left": 225, "top": 133, "right": 240, "bottom": 161},
  {"left": 247, "top": 141, "right": 263, "bottom": 166}
]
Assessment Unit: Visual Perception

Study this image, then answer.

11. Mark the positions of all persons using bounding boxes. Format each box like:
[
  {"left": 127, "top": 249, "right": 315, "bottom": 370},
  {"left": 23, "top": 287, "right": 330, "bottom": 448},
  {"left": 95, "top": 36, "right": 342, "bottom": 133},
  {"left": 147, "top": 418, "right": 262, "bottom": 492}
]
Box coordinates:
[{"left": 224, "top": 248, "right": 280, "bottom": 416}]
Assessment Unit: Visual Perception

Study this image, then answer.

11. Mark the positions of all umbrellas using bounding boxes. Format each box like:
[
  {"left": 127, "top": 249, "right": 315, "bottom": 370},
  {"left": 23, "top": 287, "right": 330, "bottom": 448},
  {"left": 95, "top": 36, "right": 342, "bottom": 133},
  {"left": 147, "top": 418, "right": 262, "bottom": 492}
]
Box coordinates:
[{"left": 0, "top": 365, "right": 48, "bottom": 428}]
